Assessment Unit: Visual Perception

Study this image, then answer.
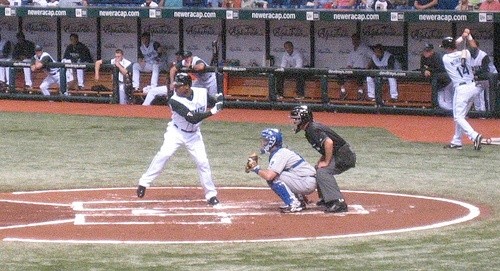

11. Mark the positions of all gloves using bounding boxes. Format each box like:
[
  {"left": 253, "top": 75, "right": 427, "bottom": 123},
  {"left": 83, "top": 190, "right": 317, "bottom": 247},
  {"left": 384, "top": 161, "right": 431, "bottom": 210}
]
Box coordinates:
[
  {"left": 210, "top": 102, "right": 224, "bottom": 115},
  {"left": 214, "top": 93, "right": 224, "bottom": 102}
]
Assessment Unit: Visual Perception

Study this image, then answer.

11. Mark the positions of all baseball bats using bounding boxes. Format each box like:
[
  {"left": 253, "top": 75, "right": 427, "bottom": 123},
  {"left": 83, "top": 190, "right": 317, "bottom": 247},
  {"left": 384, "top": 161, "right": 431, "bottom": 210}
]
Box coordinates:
[
  {"left": 212, "top": 40, "right": 220, "bottom": 94},
  {"left": 461, "top": 37, "right": 467, "bottom": 67}
]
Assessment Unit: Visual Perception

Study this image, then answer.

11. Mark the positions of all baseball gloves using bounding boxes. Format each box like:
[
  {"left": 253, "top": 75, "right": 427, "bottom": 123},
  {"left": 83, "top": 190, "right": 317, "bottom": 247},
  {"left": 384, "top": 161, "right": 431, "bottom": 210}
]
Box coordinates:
[{"left": 245, "top": 153, "right": 258, "bottom": 174}]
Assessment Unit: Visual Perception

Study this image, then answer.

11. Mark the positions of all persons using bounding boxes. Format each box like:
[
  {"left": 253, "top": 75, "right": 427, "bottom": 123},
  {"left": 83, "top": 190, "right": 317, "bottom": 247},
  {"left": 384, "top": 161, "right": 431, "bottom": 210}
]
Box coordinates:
[
  {"left": 421, "top": 41, "right": 451, "bottom": 106},
  {"left": 277, "top": 41, "right": 307, "bottom": 99},
  {"left": 63, "top": 34, "right": 93, "bottom": 87},
  {"left": 439, "top": 30, "right": 482, "bottom": 150},
  {"left": 470, "top": 40, "right": 496, "bottom": 118},
  {"left": 13, "top": 32, "right": 34, "bottom": 89},
  {"left": 169, "top": 50, "right": 222, "bottom": 100},
  {"left": 141, "top": 1, "right": 160, "bottom": 8},
  {"left": 171, "top": 51, "right": 185, "bottom": 66},
  {"left": 245, "top": 129, "right": 317, "bottom": 212},
  {"left": 143, "top": 84, "right": 171, "bottom": 105},
  {"left": 367, "top": 45, "right": 402, "bottom": 100},
  {"left": 0, "top": 33, "right": 9, "bottom": 83},
  {"left": 160, "top": 1, "right": 314, "bottom": 9},
  {"left": 290, "top": 106, "right": 355, "bottom": 214},
  {"left": 95, "top": 50, "right": 132, "bottom": 105},
  {"left": 133, "top": 33, "right": 161, "bottom": 91},
  {"left": 32, "top": 45, "right": 60, "bottom": 95},
  {"left": 337, "top": 35, "right": 375, "bottom": 101},
  {"left": 314, "top": 0, "right": 496, "bottom": 11},
  {"left": 137, "top": 73, "right": 224, "bottom": 205}
]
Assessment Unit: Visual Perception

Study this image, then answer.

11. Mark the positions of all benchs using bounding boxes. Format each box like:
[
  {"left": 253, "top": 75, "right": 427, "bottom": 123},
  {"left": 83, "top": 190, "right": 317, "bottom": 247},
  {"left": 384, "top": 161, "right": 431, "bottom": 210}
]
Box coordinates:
[{"left": 15, "top": 71, "right": 432, "bottom": 108}]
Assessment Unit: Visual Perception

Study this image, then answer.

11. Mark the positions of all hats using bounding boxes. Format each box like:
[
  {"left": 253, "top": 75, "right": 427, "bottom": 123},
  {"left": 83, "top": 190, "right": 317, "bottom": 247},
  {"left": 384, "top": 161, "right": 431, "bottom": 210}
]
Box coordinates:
[
  {"left": 182, "top": 51, "right": 192, "bottom": 58},
  {"left": 34, "top": 44, "right": 42, "bottom": 51},
  {"left": 439, "top": 37, "right": 456, "bottom": 48}
]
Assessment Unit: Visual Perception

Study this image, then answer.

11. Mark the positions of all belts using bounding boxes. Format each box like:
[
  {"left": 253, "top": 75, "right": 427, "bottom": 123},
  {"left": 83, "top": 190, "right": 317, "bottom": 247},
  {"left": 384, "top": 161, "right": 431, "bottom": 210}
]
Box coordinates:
[
  {"left": 459, "top": 80, "right": 473, "bottom": 85},
  {"left": 174, "top": 124, "right": 197, "bottom": 134},
  {"left": 53, "top": 69, "right": 60, "bottom": 75},
  {"left": 300, "top": 175, "right": 315, "bottom": 177}
]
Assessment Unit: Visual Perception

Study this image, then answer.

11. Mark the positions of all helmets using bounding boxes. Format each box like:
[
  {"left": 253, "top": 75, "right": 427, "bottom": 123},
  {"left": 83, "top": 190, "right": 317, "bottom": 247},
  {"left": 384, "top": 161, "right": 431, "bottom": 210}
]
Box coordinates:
[
  {"left": 290, "top": 105, "right": 312, "bottom": 135},
  {"left": 172, "top": 72, "right": 192, "bottom": 87},
  {"left": 258, "top": 128, "right": 283, "bottom": 155}
]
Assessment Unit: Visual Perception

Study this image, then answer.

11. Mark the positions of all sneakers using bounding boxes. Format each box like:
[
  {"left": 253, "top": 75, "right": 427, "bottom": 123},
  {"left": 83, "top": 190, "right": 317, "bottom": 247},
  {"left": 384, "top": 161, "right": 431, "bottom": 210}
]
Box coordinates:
[
  {"left": 138, "top": 185, "right": 146, "bottom": 198},
  {"left": 209, "top": 196, "right": 219, "bottom": 205},
  {"left": 325, "top": 199, "right": 348, "bottom": 213},
  {"left": 473, "top": 134, "right": 482, "bottom": 150},
  {"left": 279, "top": 203, "right": 303, "bottom": 212},
  {"left": 338, "top": 90, "right": 349, "bottom": 98},
  {"left": 443, "top": 143, "right": 462, "bottom": 149},
  {"left": 357, "top": 91, "right": 364, "bottom": 100}
]
